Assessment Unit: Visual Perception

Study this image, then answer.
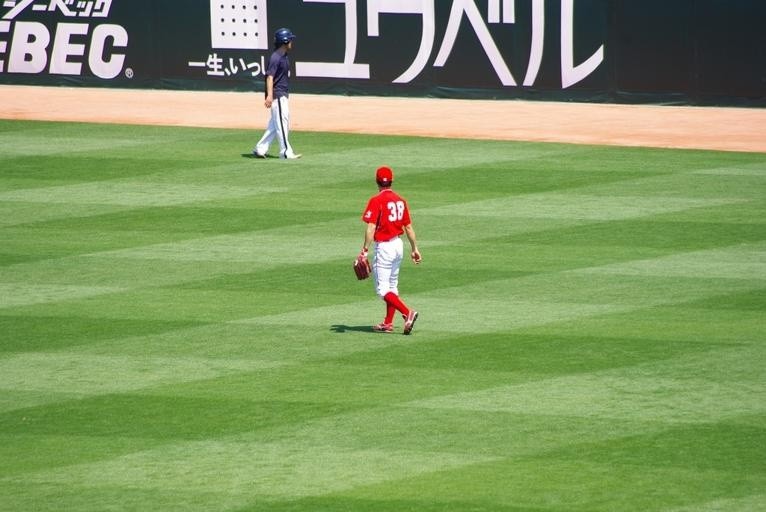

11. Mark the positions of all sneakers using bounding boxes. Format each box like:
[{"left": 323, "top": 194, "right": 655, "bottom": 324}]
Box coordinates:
[
  {"left": 403, "top": 310, "right": 418, "bottom": 335},
  {"left": 293, "top": 154, "right": 303, "bottom": 158},
  {"left": 373, "top": 322, "right": 393, "bottom": 332}
]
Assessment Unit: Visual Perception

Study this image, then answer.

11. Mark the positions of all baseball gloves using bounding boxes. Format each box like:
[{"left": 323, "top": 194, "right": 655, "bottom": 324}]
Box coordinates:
[{"left": 353, "top": 254, "right": 372, "bottom": 281}]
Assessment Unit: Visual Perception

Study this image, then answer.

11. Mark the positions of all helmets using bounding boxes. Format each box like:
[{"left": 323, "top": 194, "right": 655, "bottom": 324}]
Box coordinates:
[
  {"left": 376, "top": 167, "right": 392, "bottom": 182},
  {"left": 272, "top": 28, "right": 296, "bottom": 43}
]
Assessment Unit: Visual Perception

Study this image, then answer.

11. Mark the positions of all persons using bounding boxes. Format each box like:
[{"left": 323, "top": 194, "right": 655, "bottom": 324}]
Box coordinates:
[
  {"left": 352, "top": 166, "right": 422, "bottom": 334},
  {"left": 250, "top": 28, "right": 303, "bottom": 159}
]
